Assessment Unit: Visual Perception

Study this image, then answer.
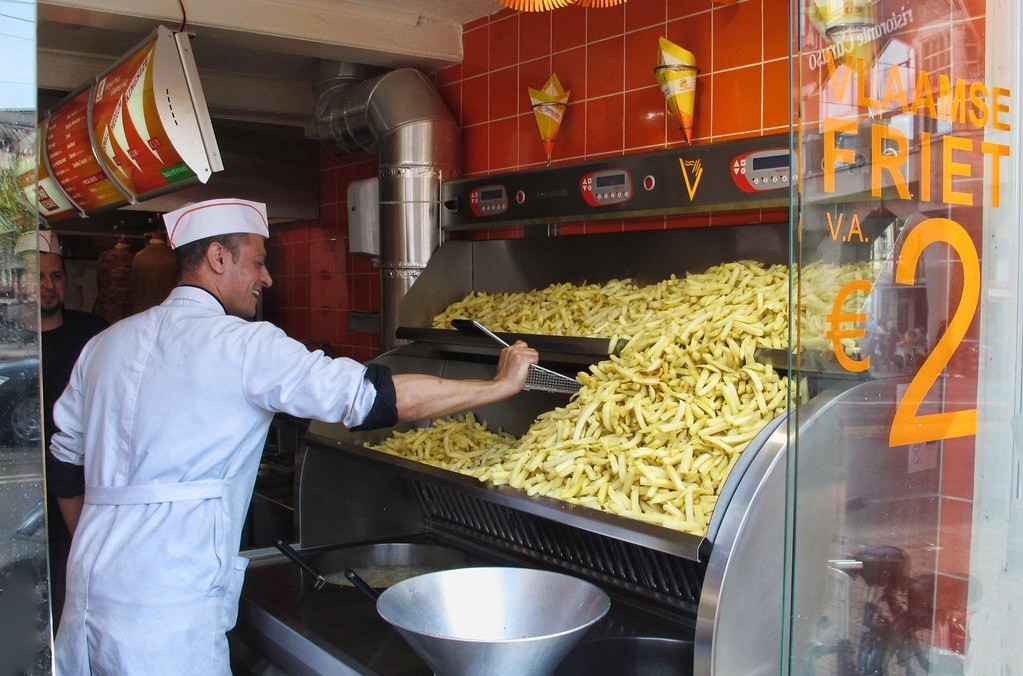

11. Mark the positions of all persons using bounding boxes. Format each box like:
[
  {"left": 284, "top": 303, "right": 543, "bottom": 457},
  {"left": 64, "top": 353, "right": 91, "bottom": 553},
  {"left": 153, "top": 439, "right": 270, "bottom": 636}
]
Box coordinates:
[
  {"left": 11, "top": 229, "right": 113, "bottom": 645},
  {"left": 48, "top": 193, "right": 539, "bottom": 675}
]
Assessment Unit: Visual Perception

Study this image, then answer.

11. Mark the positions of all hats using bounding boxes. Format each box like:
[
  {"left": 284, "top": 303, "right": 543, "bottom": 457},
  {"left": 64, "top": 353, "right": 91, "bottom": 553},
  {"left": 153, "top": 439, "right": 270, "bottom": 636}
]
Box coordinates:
[
  {"left": 15, "top": 230, "right": 63, "bottom": 255},
  {"left": 162, "top": 197, "right": 269, "bottom": 250}
]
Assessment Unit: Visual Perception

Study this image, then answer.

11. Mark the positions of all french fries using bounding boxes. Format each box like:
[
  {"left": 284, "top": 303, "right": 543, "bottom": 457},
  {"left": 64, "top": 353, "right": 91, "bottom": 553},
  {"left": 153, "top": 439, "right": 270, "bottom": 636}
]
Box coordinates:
[{"left": 363, "top": 259, "right": 888, "bottom": 538}]
